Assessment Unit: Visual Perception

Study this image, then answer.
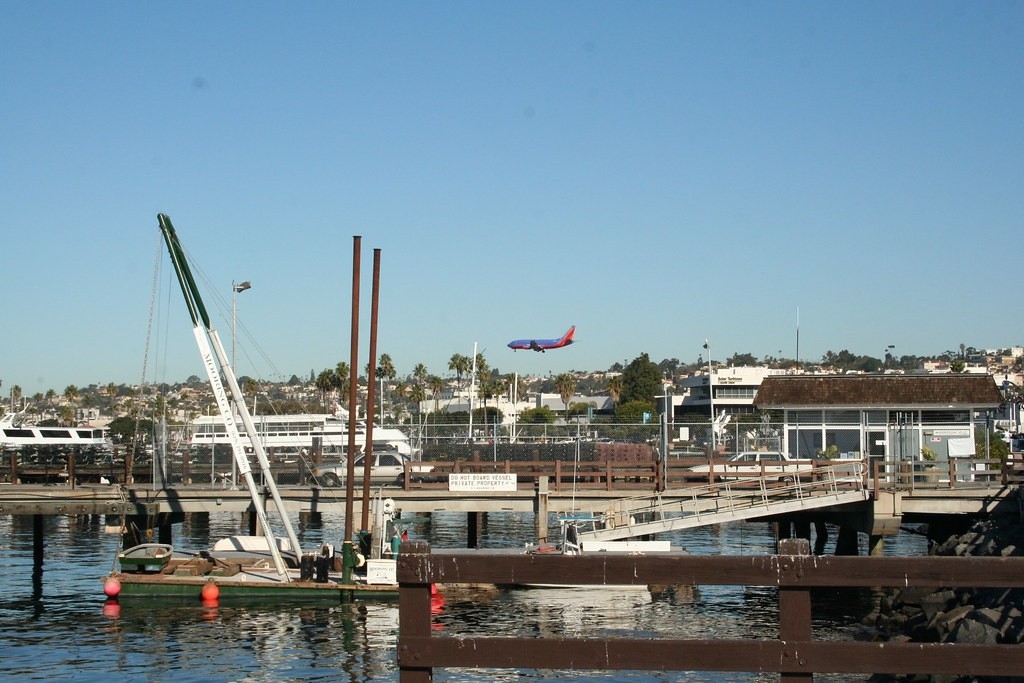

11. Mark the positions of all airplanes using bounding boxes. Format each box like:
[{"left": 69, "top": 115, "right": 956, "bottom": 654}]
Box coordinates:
[{"left": 507, "top": 325, "right": 576, "bottom": 354}]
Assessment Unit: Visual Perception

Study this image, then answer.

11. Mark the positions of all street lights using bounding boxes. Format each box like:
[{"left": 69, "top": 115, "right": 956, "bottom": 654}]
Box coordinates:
[
  {"left": 702, "top": 339, "right": 716, "bottom": 451},
  {"left": 229, "top": 280, "right": 253, "bottom": 491}
]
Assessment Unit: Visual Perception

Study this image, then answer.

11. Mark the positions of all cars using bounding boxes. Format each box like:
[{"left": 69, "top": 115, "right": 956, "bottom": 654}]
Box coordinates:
[
  {"left": 683, "top": 449, "right": 813, "bottom": 488},
  {"left": 310, "top": 449, "right": 434, "bottom": 487}
]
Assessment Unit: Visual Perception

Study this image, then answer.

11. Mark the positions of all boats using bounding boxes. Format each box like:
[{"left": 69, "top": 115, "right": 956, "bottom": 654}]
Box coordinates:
[
  {"left": 107, "top": 213, "right": 402, "bottom": 602},
  {"left": 115, "top": 541, "right": 174, "bottom": 573}
]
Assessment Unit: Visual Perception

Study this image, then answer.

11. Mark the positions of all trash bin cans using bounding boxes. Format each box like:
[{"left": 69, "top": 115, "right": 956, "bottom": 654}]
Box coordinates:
[
  {"left": 317, "top": 556, "right": 329, "bottom": 584},
  {"left": 301, "top": 555, "right": 314, "bottom": 583}
]
